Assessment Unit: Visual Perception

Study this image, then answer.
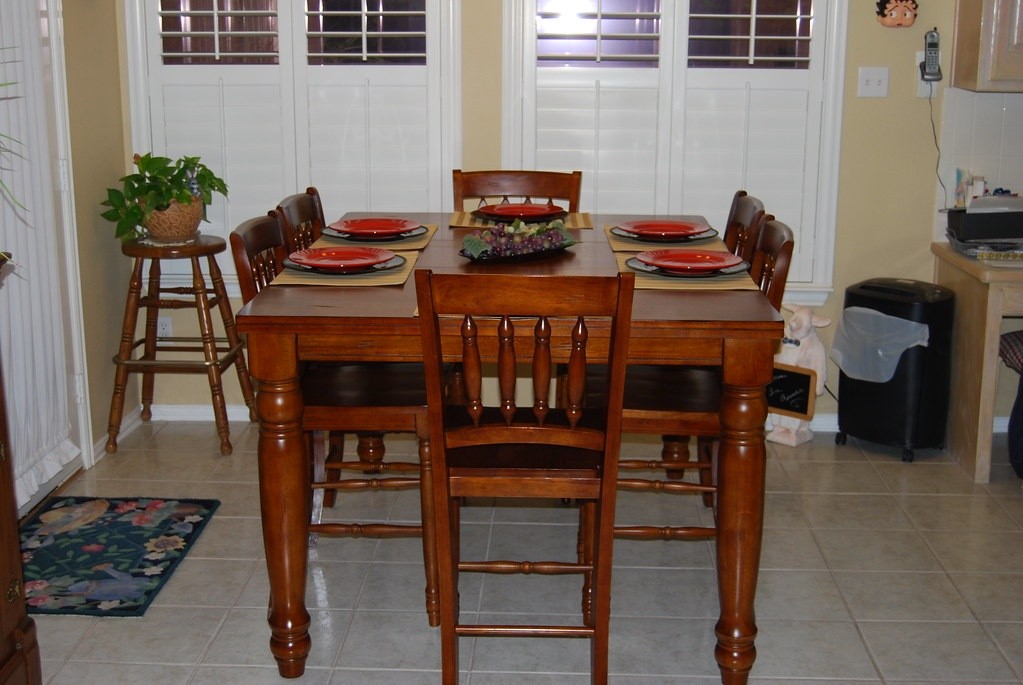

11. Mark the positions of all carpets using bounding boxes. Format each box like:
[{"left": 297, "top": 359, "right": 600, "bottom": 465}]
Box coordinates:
[{"left": 20, "top": 495, "right": 222, "bottom": 615}]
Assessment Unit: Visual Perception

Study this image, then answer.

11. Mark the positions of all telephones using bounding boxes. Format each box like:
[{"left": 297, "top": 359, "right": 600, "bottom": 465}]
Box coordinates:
[{"left": 921, "top": 25, "right": 942, "bottom": 82}]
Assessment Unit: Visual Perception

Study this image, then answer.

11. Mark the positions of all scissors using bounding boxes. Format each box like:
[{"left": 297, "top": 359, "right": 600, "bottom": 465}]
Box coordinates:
[{"left": 994, "top": 184, "right": 1009, "bottom": 195}]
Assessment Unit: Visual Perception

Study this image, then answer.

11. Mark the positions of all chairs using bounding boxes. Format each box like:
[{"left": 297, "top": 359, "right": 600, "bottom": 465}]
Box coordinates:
[
  {"left": 410, "top": 268, "right": 644, "bottom": 681},
  {"left": 445, "top": 161, "right": 593, "bottom": 501},
  {"left": 596, "top": 190, "right": 767, "bottom": 508},
  {"left": 278, "top": 178, "right": 464, "bottom": 510},
  {"left": 227, "top": 209, "right": 438, "bottom": 626},
  {"left": 614, "top": 214, "right": 802, "bottom": 510}
]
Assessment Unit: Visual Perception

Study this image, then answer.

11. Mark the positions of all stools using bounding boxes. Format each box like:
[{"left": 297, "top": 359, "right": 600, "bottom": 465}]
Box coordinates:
[
  {"left": 103, "top": 235, "right": 257, "bottom": 457},
  {"left": 999, "top": 330, "right": 1023, "bottom": 479}
]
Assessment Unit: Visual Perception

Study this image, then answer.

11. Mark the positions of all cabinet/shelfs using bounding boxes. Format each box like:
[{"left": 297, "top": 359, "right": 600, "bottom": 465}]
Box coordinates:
[{"left": 948, "top": 0, "right": 1023, "bottom": 93}]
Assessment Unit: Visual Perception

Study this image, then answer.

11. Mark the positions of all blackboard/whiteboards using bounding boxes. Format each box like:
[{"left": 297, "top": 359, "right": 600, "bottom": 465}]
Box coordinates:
[{"left": 766, "top": 362, "right": 818, "bottom": 422}]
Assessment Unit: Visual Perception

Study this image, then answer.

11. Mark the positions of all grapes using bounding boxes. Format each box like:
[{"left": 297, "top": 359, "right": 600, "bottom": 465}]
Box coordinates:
[{"left": 473, "top": 223, "right": 564, "bottom": 257}]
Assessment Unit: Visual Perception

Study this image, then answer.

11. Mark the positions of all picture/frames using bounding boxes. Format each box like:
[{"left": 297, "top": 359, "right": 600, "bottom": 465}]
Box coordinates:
[{"left": 764, "top": 363, "right": 817, "bottom": 421}]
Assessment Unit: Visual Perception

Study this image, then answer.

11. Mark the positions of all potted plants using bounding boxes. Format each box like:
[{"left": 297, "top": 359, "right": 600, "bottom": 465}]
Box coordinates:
[{"left": 99, "top": 151, "right": 231, "bottom": 239}]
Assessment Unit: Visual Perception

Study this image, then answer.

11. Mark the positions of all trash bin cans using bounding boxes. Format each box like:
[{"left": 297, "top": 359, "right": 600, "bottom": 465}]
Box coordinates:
[{"left": 835, "top": 275, "right": 955, "bottom": 462}]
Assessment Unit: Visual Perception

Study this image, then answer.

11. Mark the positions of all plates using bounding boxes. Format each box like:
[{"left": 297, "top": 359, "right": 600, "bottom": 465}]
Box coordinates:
[
  {"left": 473, "top": 203, "right": 567, "bottom": 223},
  {"left": 322, "top": 219, "right": 428, "bottom": 241},
  {"left": 282, "top": 247, "right": 407, "bottom": 275},
  {"left": 610, "top": 221, "right": 718, "bottom": 243},
  {"left": 458, "top": 239, "right": 575, "bottom": 261},
  {"left": 625, "top": 250, "right": 752, "bottom": 277}
]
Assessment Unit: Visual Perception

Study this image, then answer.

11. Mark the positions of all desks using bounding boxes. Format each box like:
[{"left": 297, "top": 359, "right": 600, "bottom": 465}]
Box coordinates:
[
  {"left": 929, "top": 240, "right": 1023, "bottom": 484},
  {"left": 234, "top": 205, "right": 786, "bottom": 681}
]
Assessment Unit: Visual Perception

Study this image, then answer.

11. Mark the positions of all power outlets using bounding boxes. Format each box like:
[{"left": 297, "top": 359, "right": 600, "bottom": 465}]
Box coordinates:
[
  {"left": 860, "top": 67, "right": 890, "bottom": 97},
  {"left": 916, "top": 51, "right": 942, "bottom": 96}
]
